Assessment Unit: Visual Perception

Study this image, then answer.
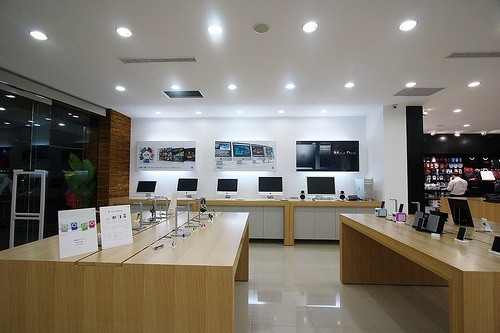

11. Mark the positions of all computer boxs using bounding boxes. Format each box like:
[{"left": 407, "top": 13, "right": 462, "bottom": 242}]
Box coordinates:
[{"left": 354, "top": 177, "right": 374, "bottom": 200}]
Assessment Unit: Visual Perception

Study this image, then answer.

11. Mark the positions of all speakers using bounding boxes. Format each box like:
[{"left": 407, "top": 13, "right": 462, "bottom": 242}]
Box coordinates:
[
  {"left": 339, "top": 190, "right": 346, "bottom": 201},
  {"left": 300, "top": 190, "right": 306, "bottom": 200}
]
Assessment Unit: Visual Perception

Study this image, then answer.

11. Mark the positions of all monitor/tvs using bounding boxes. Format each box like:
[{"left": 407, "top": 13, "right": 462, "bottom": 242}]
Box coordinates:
[
  {"left": 136, "top": 181, "right": 157, "bottom": 198},
  {"left": 176, "top": 178, "right": 199, "bottom": 198},
  {"left": 446, "top": 196, "right": 475, "bottom": 228},
  {"left": 216, "top": 179, "right": 238, "bottom": 199},
  {"left": 307, "top": 177, "right": 335, "bottom": 197},
  {"left": 258, "top": 177, "right": 282, "bottom": 200}
]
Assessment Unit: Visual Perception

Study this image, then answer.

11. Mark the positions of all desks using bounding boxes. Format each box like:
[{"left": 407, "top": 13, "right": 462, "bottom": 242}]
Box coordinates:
[{"left": 0, "top": 196, "right": 500, "bottom": 333}]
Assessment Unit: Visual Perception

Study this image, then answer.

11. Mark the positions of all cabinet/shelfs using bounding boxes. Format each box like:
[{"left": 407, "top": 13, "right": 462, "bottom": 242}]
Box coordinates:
[{"left": 423, "top": 154, "right": 464, "bottom": 192}]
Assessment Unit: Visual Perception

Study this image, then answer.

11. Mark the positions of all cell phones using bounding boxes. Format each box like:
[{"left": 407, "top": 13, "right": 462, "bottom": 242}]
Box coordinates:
[
  {"left": 490, "top": 235, "right": 500, "bottom": 253},
  {"left": 456, "top": 226, "right": 467, "bottom": 240}
]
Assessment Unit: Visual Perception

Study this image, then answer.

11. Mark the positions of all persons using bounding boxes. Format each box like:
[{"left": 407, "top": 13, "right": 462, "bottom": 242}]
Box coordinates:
[{"left": 446, "top": 172, "right": 468, "bottom": 197}]
